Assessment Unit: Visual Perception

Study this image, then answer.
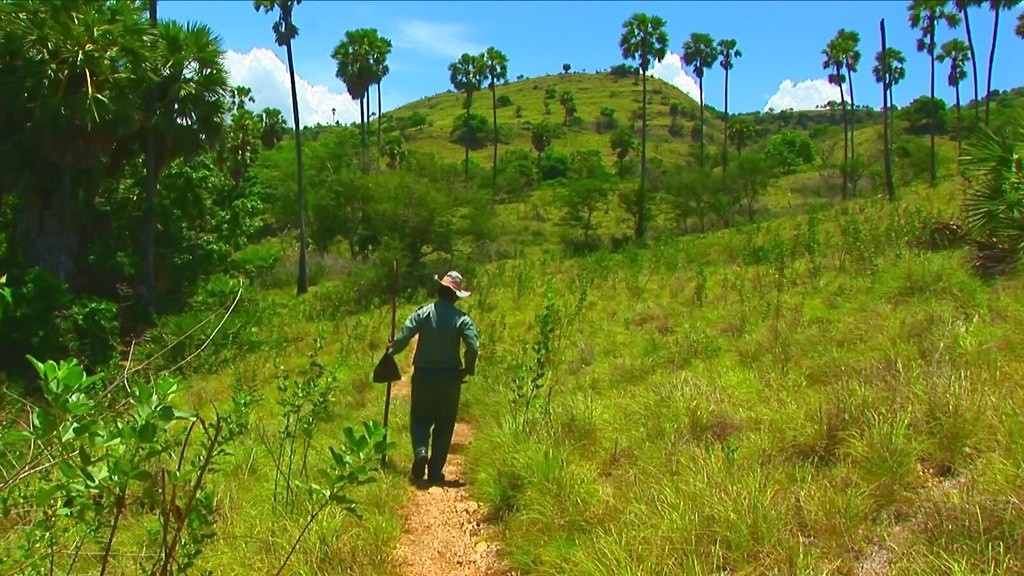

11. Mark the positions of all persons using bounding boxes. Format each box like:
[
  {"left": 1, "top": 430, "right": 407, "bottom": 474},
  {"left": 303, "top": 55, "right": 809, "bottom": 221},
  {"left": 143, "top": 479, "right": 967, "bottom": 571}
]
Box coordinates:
[
  {"left": 332, "top": 108, "right": 339, "bottom": 126},
  {"left": 386, "top": 270, "right": 478, "bottom": 485}
]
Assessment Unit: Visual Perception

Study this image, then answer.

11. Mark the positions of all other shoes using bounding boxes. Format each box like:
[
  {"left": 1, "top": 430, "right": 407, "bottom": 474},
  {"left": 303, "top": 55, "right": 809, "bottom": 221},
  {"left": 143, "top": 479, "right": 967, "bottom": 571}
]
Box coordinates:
[
  {"left": 428, "top": 472, "right": 445, "bottom": 481},
  {"left": 412, "top": 448, "right": 428, "bottom": 478}
]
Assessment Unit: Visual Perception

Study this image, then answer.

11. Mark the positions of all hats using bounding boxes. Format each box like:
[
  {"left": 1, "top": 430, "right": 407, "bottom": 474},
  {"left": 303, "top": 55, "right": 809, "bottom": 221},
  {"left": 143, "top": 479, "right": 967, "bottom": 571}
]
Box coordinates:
[{"left": 435, "top": 271, "right": 471, "bottom": 298}]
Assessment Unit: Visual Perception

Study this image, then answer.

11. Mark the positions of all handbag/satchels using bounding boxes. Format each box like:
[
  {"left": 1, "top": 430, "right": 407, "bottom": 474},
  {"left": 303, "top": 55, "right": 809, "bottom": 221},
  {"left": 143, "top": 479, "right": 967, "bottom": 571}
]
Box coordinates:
[{"left": 373, "top": 350, "right": 401, "bottom": 383}]
